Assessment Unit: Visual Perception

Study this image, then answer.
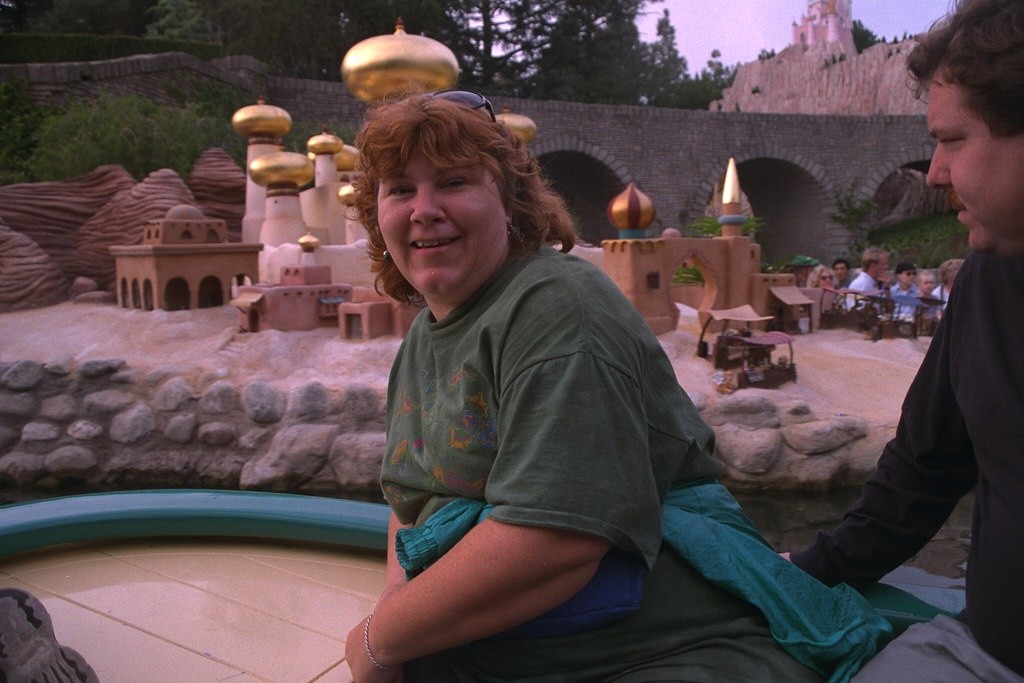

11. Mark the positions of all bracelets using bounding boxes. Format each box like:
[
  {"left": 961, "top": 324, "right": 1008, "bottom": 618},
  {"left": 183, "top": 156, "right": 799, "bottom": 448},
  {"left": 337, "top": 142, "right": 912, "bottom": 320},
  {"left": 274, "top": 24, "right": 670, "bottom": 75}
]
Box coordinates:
[{"left": 364, "top": 612, "right": 399, "bottom": 671}]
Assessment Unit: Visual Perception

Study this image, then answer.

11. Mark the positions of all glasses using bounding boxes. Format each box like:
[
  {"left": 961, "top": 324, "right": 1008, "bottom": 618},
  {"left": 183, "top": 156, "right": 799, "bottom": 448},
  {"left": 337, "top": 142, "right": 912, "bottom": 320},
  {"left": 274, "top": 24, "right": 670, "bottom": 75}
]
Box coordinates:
[
  {"left": 422, "top": 90, "right": 496, "bottom": 126},
  {"left": 821, "top": 275, "right": 834, "bottom": 280},
  {"left": 904, "top": 272, "right": 917, "bottom": 278}
]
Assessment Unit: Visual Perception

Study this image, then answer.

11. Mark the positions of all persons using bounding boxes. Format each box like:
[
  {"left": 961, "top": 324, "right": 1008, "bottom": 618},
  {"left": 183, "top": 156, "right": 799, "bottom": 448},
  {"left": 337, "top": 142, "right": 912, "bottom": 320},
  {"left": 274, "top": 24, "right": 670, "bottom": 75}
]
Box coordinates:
[
  {"left": 886, "top": 260, "right": 923, "bottom": 324},
  {"left": 806, "top": 263, "right": 834, "bottom": 308},
  {"left": 845, "top": 247, "right": 894, "bottom": 315},
  {"left": 341, "top": 91, "right": 894, "bottom": 683},
  {"left": 928, "top": 258, "right": 965, "bottom": 321},
  {"left": 831, "top": 259, "right": 850, "bottom": 307},
  {"left": 908, "top": 266, "right": 944, "bottom": 323},
  {"left": 773, "top": 0, "right": 1024, "bottom": 683}
]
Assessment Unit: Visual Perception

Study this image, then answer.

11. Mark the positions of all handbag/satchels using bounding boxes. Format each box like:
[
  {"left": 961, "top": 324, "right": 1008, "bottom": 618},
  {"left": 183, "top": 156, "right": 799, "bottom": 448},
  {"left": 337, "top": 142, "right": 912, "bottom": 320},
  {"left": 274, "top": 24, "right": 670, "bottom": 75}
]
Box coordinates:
[{"left": 483, "top": 546, "right": 646, "bottom": 640}]
